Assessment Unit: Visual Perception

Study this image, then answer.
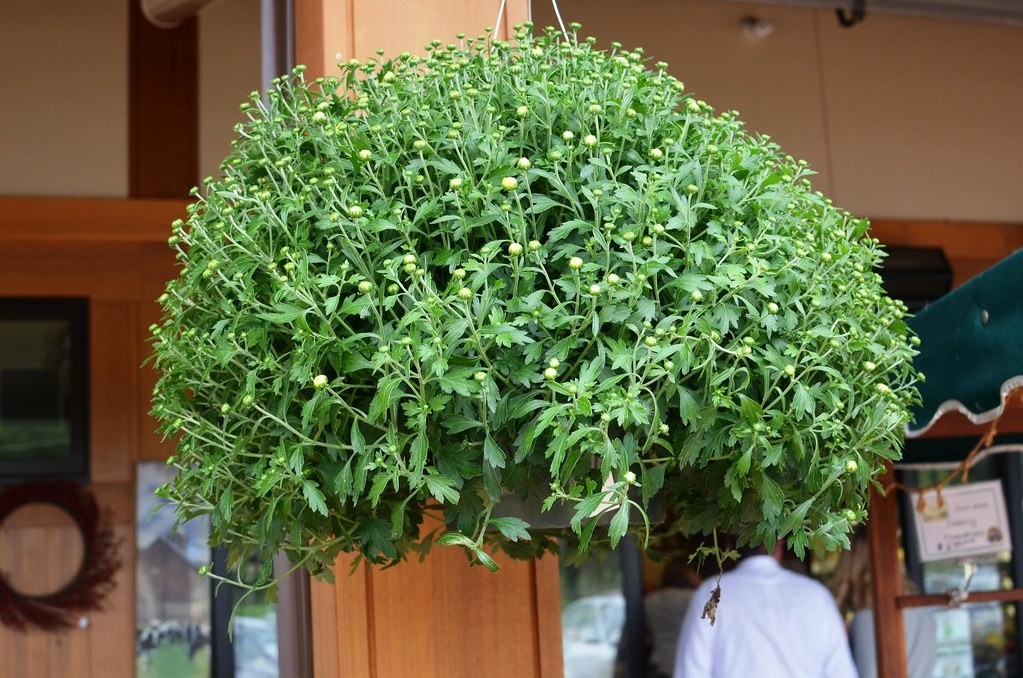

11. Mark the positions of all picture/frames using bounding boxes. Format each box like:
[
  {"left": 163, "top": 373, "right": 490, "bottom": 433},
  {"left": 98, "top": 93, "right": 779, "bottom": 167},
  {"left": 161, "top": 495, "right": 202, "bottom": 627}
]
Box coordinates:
[{"left": 1, "top": 298, "right": 89, "bottom": 482}]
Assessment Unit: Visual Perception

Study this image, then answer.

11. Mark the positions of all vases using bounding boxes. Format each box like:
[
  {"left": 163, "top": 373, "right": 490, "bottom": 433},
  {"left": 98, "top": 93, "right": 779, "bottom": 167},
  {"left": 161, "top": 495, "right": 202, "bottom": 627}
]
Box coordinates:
[{"left": 444, "top": 475, "right": 667, "bottom": 533}]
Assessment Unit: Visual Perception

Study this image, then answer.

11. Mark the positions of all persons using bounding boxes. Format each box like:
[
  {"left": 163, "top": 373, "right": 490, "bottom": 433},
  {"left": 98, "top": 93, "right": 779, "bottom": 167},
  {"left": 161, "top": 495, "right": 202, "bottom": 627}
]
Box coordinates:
[
  {"left": 643, "top": 556, "right": 696, "bottom": 678},
  {"left": 848, "top": 557, "right": 938, "bottom": 678},
  {"left": 671, "top": 534, "right": 861, "bottom": 678}
]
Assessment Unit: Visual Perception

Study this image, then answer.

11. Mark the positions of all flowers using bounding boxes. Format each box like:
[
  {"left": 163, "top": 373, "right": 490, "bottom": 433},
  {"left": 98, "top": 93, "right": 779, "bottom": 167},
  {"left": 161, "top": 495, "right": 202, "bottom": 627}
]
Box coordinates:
[{"left": 140, "top": 22, "right": 926, "bottom": 645}]
